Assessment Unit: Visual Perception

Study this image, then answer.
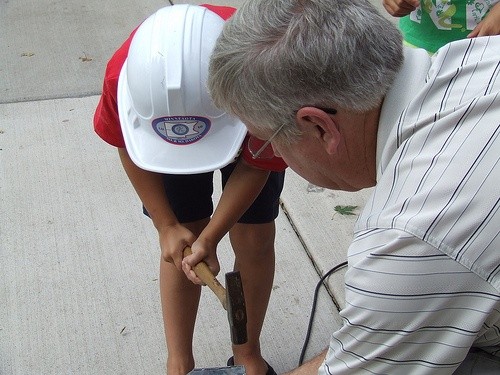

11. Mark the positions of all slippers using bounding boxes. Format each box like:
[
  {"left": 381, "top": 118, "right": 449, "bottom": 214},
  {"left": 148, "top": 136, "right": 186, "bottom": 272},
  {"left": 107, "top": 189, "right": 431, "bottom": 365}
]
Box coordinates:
[{"left": 226, "top": 353, "right": 278, "bottom": 375}]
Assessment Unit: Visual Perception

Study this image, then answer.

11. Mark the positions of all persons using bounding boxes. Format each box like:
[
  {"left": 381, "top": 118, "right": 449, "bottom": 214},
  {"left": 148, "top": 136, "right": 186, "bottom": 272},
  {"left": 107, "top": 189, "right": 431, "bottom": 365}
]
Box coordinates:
[
  {"left": 94, "top": 4, "right": 288, "bottom": 375},
  {"left": 383, "top": 0, "right": 500, "bottom": 55},
  {"left": 209, "top": 1, "right": 500, "bottom": 375}
]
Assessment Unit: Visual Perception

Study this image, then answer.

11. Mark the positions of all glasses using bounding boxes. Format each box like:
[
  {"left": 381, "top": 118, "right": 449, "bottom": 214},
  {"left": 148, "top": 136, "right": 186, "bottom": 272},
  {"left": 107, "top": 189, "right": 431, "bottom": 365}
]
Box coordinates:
[{"left": 247, "top": 105, "right": 337, "bottom": 161}]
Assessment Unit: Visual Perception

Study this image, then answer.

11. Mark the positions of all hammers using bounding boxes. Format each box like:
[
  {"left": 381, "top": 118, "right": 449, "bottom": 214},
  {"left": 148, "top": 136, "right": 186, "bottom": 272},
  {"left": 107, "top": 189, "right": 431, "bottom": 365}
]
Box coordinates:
[{"left": 183, "top": 246, "right": 248, "bottom": 345}]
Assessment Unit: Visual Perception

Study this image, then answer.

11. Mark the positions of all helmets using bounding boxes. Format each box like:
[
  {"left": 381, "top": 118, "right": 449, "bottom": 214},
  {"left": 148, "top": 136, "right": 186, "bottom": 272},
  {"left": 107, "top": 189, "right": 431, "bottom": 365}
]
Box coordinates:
[{"left": 117, "top": 3, "right": 248, "bottom": 175}]
